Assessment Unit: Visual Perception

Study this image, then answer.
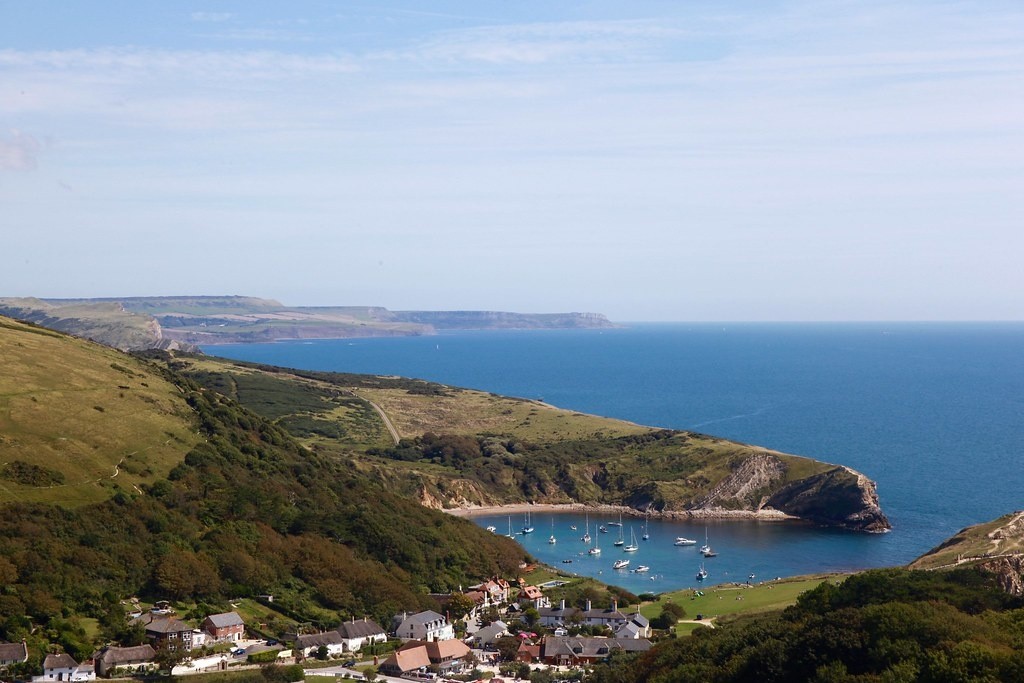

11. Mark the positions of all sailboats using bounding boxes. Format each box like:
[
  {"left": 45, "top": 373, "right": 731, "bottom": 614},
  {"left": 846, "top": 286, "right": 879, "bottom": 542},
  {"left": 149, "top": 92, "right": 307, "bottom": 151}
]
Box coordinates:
[{"left": 486, "top": 503, "right": 719, "bottom": 580}]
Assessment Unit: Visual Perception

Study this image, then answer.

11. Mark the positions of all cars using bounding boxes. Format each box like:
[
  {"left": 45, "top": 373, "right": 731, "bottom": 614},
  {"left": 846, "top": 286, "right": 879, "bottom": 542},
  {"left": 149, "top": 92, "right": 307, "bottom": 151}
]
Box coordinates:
[
  {"left": 267, "top": 639, "right": 278, "bottom": 646},
  {"left": 234, "top": 648, "right": 246, "bottom": 655},
  {"left": 342, "top": 661, "right": 356, "bottom": 668}
]
